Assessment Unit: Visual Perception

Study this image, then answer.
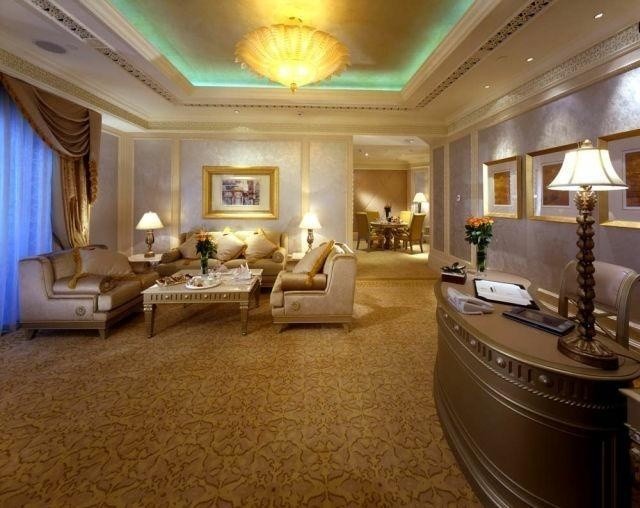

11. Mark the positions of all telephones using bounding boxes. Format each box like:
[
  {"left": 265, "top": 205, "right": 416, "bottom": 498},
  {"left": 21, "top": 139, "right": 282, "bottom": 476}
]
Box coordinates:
[{"left": 447, "top": 287, "right": 495, "bottom": 315}]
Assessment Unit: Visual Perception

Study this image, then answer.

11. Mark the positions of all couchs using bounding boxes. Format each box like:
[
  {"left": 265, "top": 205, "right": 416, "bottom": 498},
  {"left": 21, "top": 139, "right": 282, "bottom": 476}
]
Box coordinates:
[
  {"left": 16, "top": 244, "right": 160, "bottom": 340},
  {"left": 269, "top": 240, "right": 356, "bottom": 327},
  {"left": 160, "top": 228, "right": 289, "bottom": 289}
]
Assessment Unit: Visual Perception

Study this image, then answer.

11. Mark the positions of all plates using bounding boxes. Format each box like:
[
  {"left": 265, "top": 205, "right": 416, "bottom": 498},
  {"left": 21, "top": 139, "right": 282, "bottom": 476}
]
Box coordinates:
[
  {"left": 155, "top": 273, "right": 192, "bottom": 286},
  {"left": 184, "top": 282, "right": 213, "bottom": 290}
]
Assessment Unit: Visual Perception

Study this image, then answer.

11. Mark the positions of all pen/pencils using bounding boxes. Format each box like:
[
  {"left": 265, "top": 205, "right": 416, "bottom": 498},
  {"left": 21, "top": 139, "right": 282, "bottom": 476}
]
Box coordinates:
[{"left": 490, "top": 286, "right": 493, "bottom": 292}]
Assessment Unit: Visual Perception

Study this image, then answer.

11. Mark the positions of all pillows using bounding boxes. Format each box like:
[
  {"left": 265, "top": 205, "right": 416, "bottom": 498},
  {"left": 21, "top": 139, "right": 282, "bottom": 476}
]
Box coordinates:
[
  {"left": 212, "top": 232, "right": 247, "bottom": 262},
  {"left": 244, "top": 228, "right": 278, "bottom": 263},
  {"left": 178, "top": 236, "right": 201, "bottom": 259},
  {"left": 292, "top": 240, "right": 334, "bottom": 274},
  {"left": 67, "top": 246, "right": 136, "bottom": 289}
]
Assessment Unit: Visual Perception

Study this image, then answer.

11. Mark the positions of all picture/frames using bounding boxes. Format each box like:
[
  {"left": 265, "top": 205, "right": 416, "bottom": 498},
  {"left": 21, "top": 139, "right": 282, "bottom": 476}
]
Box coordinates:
[
  {"left": 597, "top": 128, "right": 639, "bottom": 229},
  {"left": 525, "top": 141, "right": 583, "bottom": 224},
  {"left": 201, "top": 162, "right": 279, "bottom": 218},
  {"left": 481, "top": 155, "right": 523, "bottom": 223}
]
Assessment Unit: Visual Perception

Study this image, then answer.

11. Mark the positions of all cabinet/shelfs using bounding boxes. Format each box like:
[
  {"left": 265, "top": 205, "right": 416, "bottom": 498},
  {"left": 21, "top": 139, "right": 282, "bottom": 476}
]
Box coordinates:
[{"left": 287, "top": 253, "right": 305, "bottom": 270}]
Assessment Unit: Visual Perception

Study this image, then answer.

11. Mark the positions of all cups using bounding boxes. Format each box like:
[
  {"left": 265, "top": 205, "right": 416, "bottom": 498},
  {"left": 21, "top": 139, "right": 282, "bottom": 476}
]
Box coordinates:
[{"left": 388, "top": 216, "right": 400, "bottom": 224}]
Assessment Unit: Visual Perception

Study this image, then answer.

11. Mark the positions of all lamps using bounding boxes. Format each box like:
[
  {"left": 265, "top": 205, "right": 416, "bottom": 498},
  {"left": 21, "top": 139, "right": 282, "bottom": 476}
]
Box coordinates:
[
  {"left": 412, "top": 191, "right": 428, "bottom": 213},
  {"left": 547, "top": 138, "right": 629, "bottom": 372},
  {"left": 135, "top": 209, "right": 164, "bottom": 257},
  {"left": 300, "top": 209, "right": 322, "bottom": 253},
  {"left": 233, "top": 24, "right": 349, "bottom": 94}
]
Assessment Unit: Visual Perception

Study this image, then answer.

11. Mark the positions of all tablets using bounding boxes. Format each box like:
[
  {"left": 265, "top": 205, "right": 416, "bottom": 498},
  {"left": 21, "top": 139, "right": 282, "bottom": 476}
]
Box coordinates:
[{"left": 503, "top": 308, "right": 575, "bottom": 337}]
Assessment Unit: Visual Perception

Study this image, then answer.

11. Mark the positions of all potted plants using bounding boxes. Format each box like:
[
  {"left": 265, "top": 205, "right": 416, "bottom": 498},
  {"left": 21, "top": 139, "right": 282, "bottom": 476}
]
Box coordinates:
[{"left": 195, "top": 238, "right": 218, "bottom": 275}]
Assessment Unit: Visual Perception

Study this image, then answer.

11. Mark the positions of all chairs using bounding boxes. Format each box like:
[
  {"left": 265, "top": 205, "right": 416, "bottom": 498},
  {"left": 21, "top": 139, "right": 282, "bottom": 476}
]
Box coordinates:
[
  {"left": 356, "top": 206, "right": 428, "bottom": 254},
  {"left": 556, "top": 257, "right": 640, "bottom": 350}
]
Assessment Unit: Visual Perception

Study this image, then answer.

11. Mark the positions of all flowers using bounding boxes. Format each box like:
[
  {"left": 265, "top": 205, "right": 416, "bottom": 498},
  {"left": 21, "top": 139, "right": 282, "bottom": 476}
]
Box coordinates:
[{"left": 462, "top": 207, "right": 494, "bottom": 246}]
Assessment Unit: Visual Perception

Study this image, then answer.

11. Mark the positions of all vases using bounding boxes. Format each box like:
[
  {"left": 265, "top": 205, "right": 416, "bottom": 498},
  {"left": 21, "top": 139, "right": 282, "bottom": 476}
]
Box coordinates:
[{"left": 477, "top": 244, "right": 487, "bottom": 277}]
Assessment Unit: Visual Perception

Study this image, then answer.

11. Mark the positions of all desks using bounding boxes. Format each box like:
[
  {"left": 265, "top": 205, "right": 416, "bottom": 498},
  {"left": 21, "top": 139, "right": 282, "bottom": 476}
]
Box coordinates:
[{"left": 423, "top": 270, "right": 639, "bottom": 507}]
